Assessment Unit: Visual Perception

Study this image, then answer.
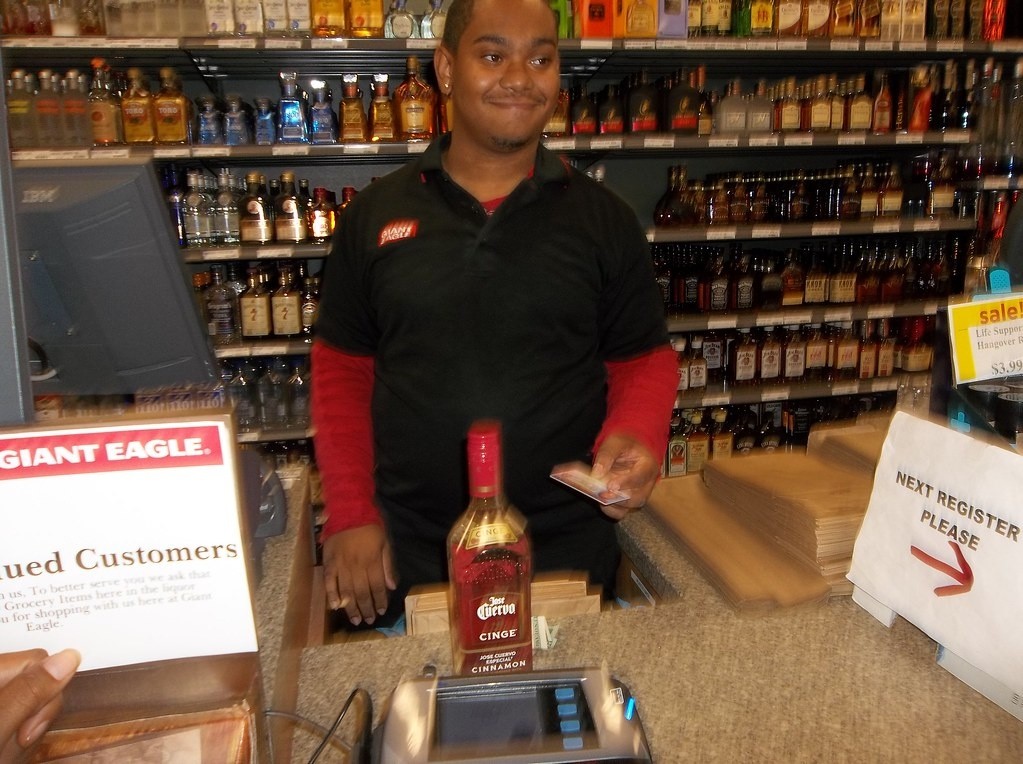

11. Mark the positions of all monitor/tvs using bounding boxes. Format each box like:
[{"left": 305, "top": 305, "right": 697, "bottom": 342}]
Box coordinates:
[{"left": 6, "top": 147, "right": 225, "bottom": 398}]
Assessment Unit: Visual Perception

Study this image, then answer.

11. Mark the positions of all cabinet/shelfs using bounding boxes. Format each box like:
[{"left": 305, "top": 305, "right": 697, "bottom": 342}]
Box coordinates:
[{"left": 2, "top": 35, "right": 1023, "bottom": 444}]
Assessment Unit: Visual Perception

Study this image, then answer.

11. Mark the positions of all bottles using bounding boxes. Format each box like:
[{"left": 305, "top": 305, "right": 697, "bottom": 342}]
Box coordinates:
[
  {"left": 255, "top": 440, "right": 323, "bottom": 512},
  {"left": 0, "top": 1, "right": 457, "bottom": 142},
  {"left": 446, "top": 421, "right": 533, "bottom": 675},
  {"left": 213, "top": 357, "right": 314, "bottom": 430},
  {"left": 165, "top": 166, "right": 380, "bottom": 248},
  {"left": 191, "top": 262, "right": 323, "bottom": 346},
  {"left": 542, "top": 0, "right": 1023, "bottom": 390},
  {"left": 656, "top": 394, "right": 894, "bottom": 482}
]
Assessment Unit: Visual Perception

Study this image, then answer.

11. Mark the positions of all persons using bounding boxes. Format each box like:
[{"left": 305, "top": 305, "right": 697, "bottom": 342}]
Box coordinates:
[
  {"left": 597, "top": 490, "right": 618, "bottom": 499},
  {"left": 311, "top": 0, "right": 679, "bottom": 635}
]
